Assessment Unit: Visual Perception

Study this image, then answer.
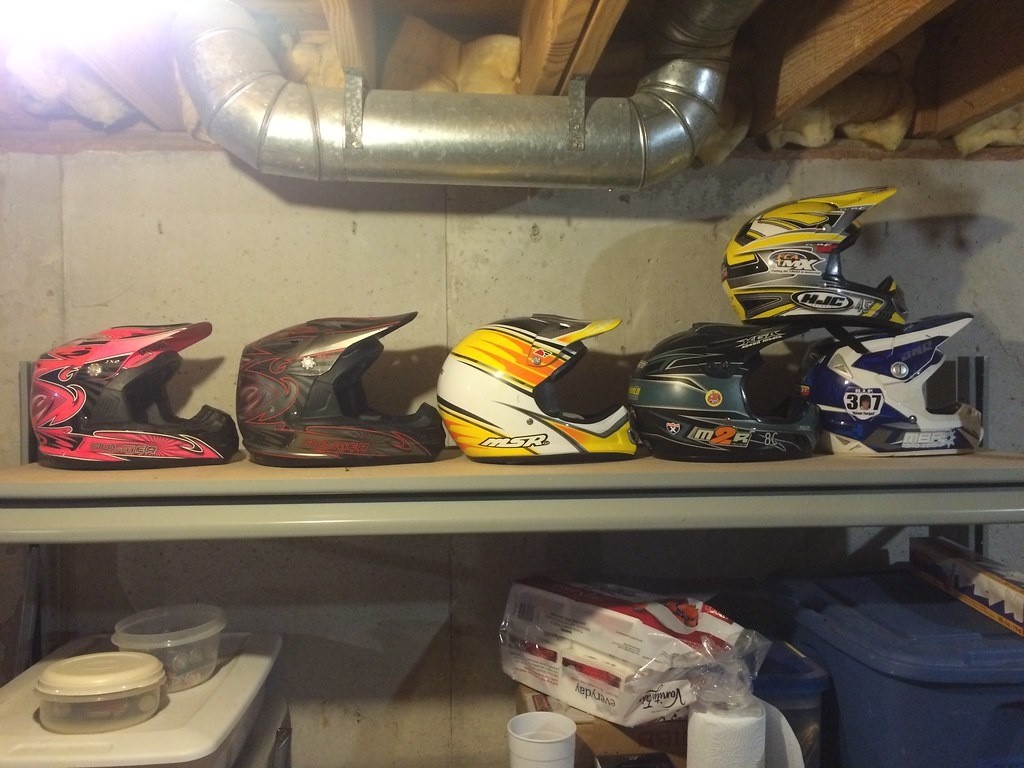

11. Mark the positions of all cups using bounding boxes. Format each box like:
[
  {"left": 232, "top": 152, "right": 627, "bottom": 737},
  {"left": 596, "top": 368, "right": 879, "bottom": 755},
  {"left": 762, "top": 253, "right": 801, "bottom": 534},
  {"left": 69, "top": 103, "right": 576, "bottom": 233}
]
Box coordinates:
[{"left": 507, "top": 711, "right": 577, "bottom": 768}]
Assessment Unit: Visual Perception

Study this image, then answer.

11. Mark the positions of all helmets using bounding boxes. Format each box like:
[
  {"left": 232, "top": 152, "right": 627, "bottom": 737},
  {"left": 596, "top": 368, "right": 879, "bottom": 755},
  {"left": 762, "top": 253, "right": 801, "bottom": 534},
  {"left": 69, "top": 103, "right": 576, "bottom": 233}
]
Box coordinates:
[
  {"left": 238, "top": 311, "right": 446, "bottom": 467},
  {"left": 29, "top": 321, "right": 239, "bottom": 471},
  {"left": 720, "top": 186, "right": 910, "bottom": 332},
  {"left": 799, "top": 312, "right": 984, "bottom": 457},
  {"left": 435, "top": 313, "right": 652, "bottom": 465},
  {"left": 626, "top": 321, "right": 823, "bottom": 460}
]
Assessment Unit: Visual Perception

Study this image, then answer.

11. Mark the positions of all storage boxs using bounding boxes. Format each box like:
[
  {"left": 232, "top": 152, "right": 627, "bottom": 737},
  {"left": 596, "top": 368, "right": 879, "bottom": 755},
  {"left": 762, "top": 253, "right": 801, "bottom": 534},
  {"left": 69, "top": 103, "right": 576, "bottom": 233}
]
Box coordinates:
[
  {"left": 513, "top": 536, "right": 1024, "bottom": 768},
  {"left": 0, "top": 625, "right": 294, "bottom": 768}
]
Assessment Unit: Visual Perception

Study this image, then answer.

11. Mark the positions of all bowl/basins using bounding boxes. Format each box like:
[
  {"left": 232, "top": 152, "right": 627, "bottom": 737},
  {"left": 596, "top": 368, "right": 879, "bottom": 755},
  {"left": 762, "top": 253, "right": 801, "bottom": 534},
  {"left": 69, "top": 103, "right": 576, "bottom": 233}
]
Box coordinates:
[
  {"left": 110, "top": 603, "right": 228, "bottom": 694},
  {"left": 33, "top": 651, "right": 167, "bottom": 734}
]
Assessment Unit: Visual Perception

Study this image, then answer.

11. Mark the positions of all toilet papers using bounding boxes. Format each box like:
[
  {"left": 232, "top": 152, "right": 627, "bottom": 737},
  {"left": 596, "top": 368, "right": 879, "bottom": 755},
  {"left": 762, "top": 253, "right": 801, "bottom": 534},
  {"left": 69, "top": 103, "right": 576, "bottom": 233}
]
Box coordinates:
[{"left": 686, "top": 693, "right": 806, "bottom": 768}]
